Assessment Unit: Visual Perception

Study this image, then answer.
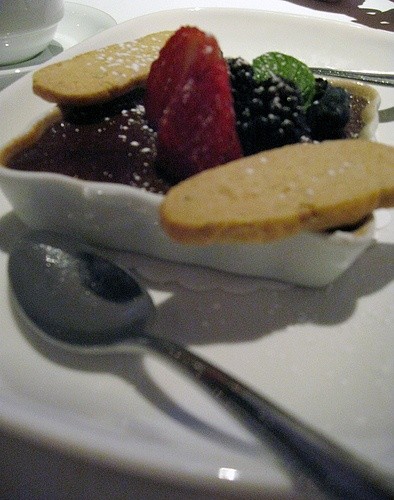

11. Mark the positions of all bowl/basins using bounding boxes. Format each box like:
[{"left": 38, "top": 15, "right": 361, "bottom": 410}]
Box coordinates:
[{"left": 0, "top": 0, "right": 66, "bottom": 66}]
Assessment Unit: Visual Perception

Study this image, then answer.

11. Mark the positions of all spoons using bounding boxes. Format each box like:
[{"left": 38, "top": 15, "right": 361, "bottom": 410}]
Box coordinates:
[{"left": 7, "top": 232, "right": 393, "bottom": 500}]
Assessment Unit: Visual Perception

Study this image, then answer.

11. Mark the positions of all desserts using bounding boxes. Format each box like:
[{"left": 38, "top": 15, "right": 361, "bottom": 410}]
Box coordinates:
[{"left": 0, "top": 25, "right": 393, "bottom": 244}]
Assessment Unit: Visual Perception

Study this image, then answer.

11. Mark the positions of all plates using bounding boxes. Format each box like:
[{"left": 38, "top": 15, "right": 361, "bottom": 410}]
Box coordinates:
[
  {"left": 0, "top": 4, "right": 118, "bottom": 113},
  {"left": 2, "top": 187, "right": 393, "bottom": 500},
  {"left": 1, "top": 2, "right": 394, "bottom": 288}
]
